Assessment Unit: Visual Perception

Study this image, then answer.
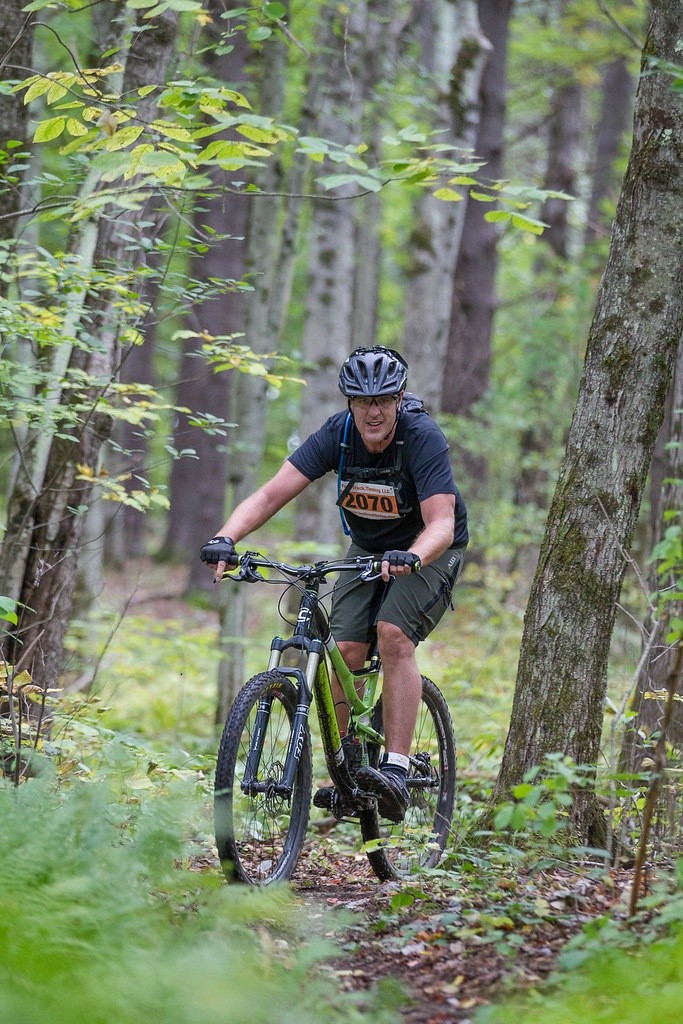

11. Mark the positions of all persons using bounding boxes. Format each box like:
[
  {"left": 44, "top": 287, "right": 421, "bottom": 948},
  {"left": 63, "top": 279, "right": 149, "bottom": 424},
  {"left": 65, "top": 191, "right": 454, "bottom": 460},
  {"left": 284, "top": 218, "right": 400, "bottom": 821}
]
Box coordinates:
[{"left": 198, "top": 346, "right": 470, "bottom": 823}]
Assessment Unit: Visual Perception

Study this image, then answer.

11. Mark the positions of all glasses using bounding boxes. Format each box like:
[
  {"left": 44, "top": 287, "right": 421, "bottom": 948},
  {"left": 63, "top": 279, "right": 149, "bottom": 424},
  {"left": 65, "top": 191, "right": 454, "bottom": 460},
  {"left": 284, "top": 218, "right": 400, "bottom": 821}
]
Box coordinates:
[{"left": 353, "top": 396, "right": 396, "bottom": 409}]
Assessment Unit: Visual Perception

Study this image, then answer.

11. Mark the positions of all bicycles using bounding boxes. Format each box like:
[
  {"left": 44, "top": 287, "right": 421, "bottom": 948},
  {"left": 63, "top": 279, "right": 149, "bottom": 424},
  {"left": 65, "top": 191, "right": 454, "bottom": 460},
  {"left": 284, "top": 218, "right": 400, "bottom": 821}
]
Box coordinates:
[{"left": 195, "top": 550, "right": 457, "bottom": 898}]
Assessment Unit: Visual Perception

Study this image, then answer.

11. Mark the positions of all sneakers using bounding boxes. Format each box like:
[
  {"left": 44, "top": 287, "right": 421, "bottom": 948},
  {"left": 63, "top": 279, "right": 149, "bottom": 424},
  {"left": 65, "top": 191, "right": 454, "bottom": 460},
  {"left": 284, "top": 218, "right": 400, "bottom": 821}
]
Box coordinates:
[
  {"left": 312, "top": 786, "right": 354, "bottom": 818},
  {"left": 356, "top": 762, "right": 409, "bottom": 821}
]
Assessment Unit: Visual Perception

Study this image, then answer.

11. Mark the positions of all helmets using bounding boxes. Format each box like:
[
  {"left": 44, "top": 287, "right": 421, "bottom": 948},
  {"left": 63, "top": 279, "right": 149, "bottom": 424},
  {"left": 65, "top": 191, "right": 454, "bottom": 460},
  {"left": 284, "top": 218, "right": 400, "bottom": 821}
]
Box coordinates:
[{"left": 338, "top": 345, "right": 409, "bottom": 396}]
